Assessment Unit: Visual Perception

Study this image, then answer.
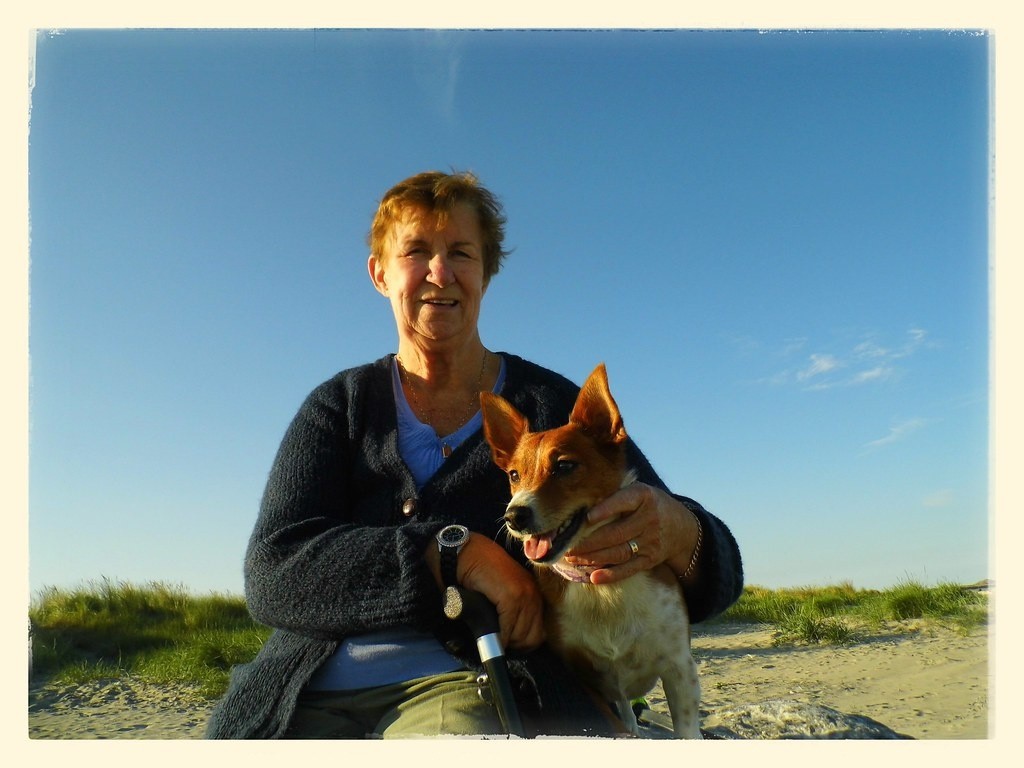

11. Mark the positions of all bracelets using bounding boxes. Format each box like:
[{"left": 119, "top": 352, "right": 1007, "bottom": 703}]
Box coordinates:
[{"left": 679, "top": 512, "right": 701, "bottom": 578}]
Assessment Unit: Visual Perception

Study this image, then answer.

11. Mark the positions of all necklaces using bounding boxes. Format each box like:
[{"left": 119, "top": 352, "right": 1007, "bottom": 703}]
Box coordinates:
[{"left": 399, "top": 350, "right": 487, "bottom": 459}]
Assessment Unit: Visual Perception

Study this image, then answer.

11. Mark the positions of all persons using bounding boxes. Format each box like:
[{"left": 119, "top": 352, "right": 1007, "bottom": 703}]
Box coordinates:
[{"left": 205, "top": 171, "right": 743, "bottom": 739}]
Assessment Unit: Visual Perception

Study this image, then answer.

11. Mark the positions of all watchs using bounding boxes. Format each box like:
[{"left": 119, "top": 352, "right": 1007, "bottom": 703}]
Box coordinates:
[{"left": 435, "top": 525, "right": 469, "bottom": 586}]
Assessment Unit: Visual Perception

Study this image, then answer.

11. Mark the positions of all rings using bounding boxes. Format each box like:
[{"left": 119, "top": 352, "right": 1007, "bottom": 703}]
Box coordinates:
[{"left": 627, "top": 540, "right": 639, "bottom": 560}]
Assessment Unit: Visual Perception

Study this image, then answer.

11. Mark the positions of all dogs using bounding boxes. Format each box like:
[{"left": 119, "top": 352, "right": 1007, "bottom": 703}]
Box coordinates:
[{"left": 479, "top": 360, "right": 702, "bottom": 740}]
